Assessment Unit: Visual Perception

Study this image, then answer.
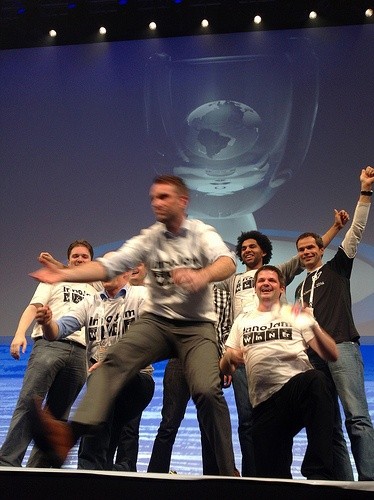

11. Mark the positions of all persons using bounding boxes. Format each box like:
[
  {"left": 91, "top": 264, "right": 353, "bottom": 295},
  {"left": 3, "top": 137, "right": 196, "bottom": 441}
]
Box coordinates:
[
  {"left": 35, "top": 251, "right": 155, "bottom": 472},
  {"left": 219, "top": 265, "right": 346, "bottom": 481},
  {"left": 213, "top": 208, "right": 349, "bottom": 477},
  {"left": 29, "top": 176, "right": 237, "bottom": 476},
  {"left": 146, "top": 284, "right": 240, "bottom": 478},
  {"left": 0, "top": 240, "right": 99, "bottom": 468},
  {"left": 291, "top": 166, "right": 374, "bottom": 482}
]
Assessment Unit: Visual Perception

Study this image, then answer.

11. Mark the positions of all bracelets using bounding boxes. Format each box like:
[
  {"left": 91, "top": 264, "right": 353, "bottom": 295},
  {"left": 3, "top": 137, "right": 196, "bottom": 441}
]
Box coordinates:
[{"left": 361, "top": 190, "right": 373, "bottom": 195}]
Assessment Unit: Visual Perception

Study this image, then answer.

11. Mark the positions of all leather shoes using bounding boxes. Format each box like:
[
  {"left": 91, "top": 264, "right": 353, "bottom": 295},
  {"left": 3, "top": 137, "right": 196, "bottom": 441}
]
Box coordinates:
[{"left": 28, "top": 395, "right": 75, "bottom": 468}]
